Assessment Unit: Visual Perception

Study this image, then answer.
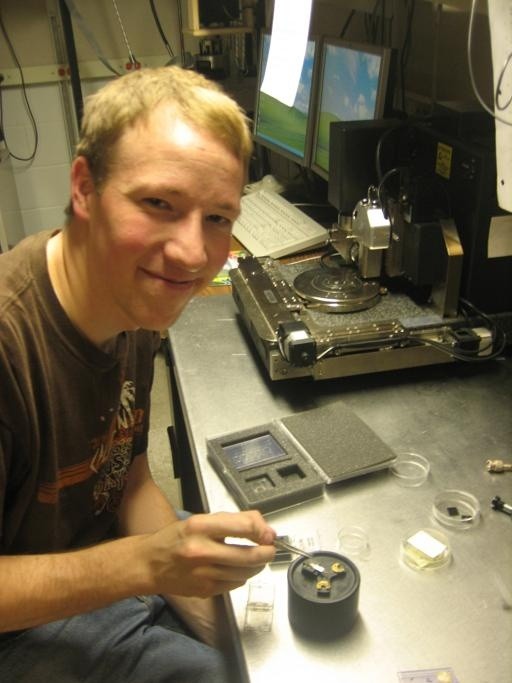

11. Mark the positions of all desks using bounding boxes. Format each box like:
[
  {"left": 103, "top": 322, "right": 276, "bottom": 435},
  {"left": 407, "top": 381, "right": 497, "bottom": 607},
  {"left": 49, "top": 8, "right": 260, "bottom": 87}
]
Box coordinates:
[{"left": 166, "top": 294, "right": 511, "bottom": 683}]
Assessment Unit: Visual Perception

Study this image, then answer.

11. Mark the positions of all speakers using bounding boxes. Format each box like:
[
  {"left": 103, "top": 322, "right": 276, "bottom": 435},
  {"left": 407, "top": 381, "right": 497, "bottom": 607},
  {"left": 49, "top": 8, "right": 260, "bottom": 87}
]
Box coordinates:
[{"left": 328, "top": 117, "right": 409, "bottom": 210}]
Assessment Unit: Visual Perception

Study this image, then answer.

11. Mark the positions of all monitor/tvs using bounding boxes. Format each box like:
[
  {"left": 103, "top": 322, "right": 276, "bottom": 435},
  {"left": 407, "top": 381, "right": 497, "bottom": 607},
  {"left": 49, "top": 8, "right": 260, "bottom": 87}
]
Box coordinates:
[
  {"left": 310, "top": 36, "right": 392, "bottom": 181},
  {"left": 252, "top": 26, "right": 323, "bottom": 168}
]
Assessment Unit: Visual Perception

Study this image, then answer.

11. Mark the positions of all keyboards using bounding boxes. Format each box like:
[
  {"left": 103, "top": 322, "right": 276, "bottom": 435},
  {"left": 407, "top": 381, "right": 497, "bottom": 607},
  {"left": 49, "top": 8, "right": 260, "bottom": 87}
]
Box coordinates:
[{"left": 232, "top": 188, "right": 330, "bottom": 259}]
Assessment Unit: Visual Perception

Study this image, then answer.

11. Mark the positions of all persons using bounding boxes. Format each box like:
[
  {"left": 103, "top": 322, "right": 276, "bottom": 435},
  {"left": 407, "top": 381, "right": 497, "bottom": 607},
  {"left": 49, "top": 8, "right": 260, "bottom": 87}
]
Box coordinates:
[{"left": 0, "top": 65, "right": 278, "bottom": 683}]
